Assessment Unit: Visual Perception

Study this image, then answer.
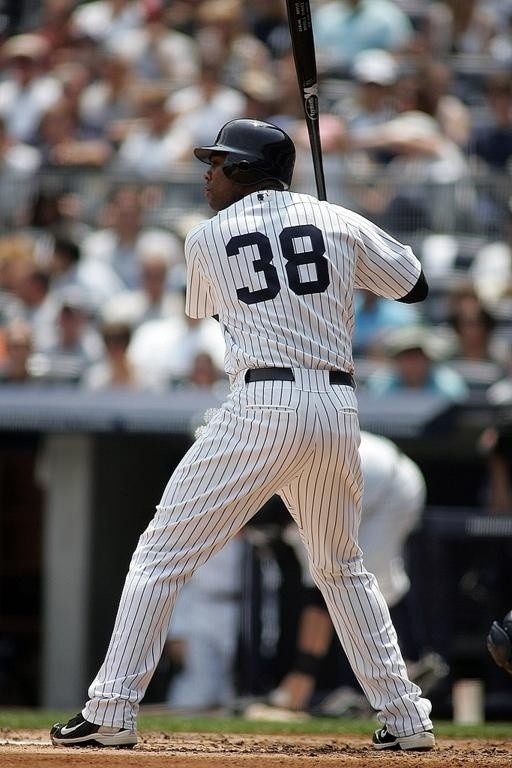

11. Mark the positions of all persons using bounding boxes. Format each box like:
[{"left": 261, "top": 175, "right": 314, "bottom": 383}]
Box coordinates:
[
  {"left": 148, "top": 397, "right": 512, "bottom": 724},
  {"left": 0, "top": 0, "right": 512, "bottom": 393},
  {"left": 46, "top": 117, "right": 438, "bottom": 748}
]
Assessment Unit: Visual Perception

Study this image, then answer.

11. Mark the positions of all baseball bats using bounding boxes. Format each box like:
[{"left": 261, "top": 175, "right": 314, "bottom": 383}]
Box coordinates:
[{"left": 286, "top": 1, "right": 327, "bottom": 202}]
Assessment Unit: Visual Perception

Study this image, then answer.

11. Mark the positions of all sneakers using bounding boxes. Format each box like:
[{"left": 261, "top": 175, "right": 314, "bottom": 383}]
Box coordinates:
[
  {"left": 371, "top": 722, "right": 436, "bottom": 751},
  {"left": 49, "top": 711, "right": 139, "bottom": 747}
]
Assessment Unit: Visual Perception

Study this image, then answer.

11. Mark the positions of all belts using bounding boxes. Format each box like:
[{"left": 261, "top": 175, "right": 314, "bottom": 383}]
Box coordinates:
[{"left": 242, "top": 366, "right": 355, "bottom": 386}]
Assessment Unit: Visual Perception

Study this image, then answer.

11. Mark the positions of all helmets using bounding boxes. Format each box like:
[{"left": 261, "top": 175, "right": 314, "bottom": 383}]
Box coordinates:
[{"left": 193, "top": 117, "right": 298, "bottom": 189}]
